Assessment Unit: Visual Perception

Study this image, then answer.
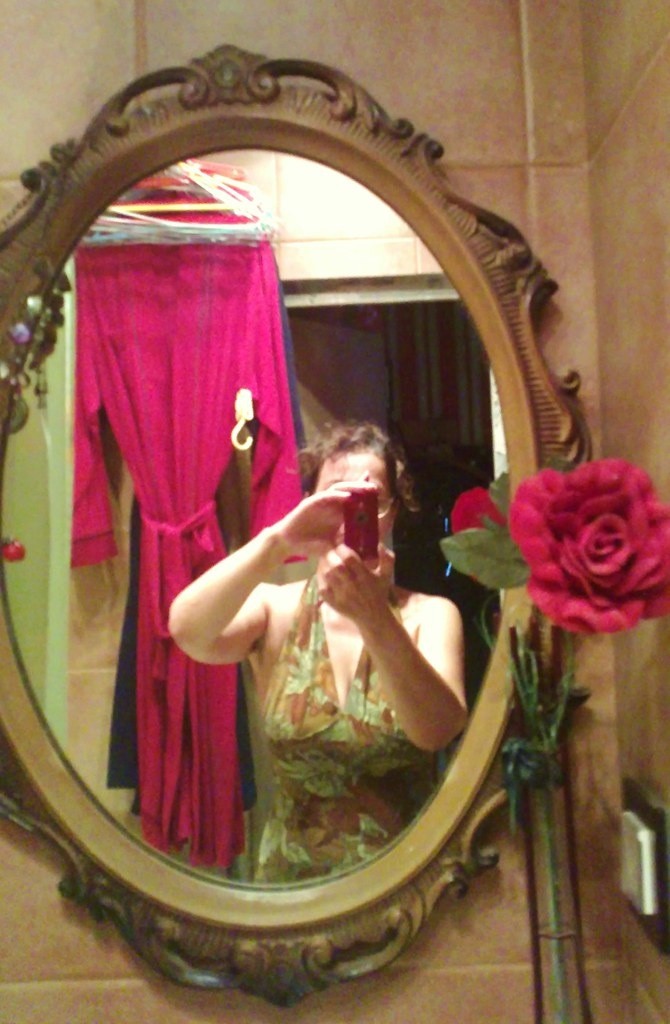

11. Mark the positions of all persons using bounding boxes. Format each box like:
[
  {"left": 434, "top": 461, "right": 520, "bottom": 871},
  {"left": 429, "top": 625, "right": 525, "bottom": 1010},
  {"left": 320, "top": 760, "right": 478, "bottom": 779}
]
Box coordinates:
[{"left": 167, "top": 419, "right": 468, "bottom": 890}]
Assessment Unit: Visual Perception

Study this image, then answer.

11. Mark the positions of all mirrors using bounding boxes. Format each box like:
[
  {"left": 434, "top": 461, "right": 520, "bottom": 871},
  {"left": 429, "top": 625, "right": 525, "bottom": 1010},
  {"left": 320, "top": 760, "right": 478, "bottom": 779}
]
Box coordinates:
[{"left": 5, "top": 43, "right": 590, "bottom": 1011}]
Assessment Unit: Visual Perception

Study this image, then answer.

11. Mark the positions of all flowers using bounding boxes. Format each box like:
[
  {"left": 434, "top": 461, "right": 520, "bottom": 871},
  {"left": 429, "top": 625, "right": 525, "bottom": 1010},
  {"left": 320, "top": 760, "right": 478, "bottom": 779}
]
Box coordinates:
[
  {"left": 446, "top": 486, "right": 527, "bottom": 588},
  {"left": 502, "top": 455, "right": 670, "bottom": 770}
]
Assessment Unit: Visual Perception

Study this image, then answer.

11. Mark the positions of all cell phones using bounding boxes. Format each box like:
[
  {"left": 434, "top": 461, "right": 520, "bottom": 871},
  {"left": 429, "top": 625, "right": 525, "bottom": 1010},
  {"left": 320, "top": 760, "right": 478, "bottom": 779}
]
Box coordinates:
[{"left": 343, "top": 493, "right": 379, "bottom": 569}]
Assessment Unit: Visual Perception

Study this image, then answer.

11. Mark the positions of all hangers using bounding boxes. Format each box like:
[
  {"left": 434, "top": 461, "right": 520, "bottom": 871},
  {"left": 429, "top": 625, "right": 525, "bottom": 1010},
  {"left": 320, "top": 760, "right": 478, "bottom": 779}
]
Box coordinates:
[{"left": 72, "top": 155, "right": 276, "bottom": 247}]
